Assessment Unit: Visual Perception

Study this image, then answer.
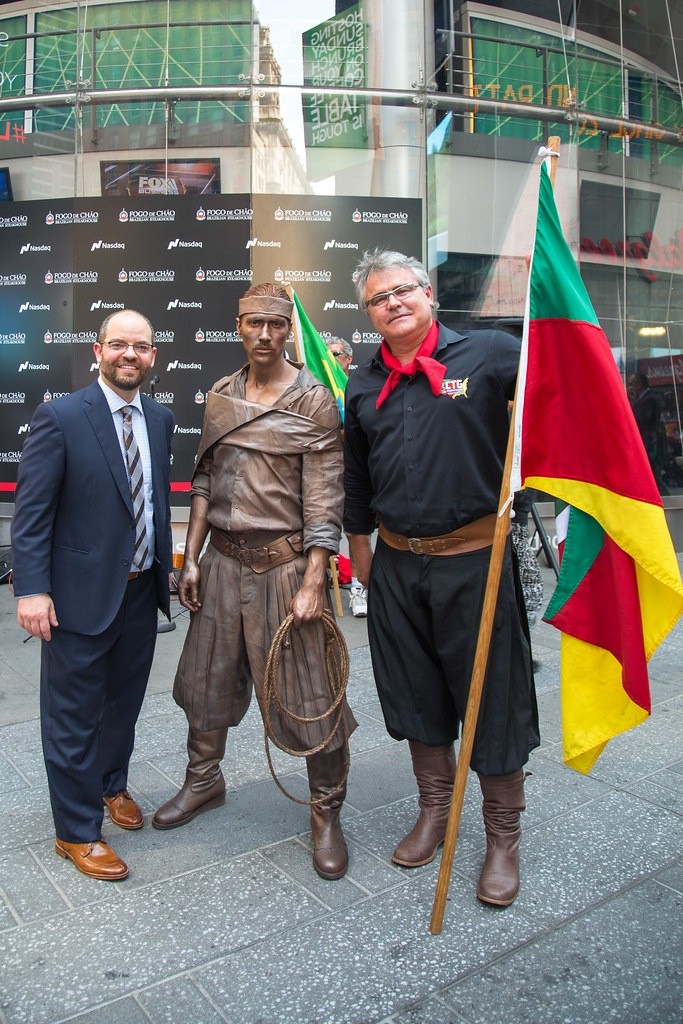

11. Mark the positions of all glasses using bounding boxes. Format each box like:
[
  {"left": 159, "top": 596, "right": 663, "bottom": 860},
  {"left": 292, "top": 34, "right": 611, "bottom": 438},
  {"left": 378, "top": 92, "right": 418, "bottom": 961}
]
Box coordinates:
[
  {"left": 364, "top": 282, "right": 421, "bottom": 311},
  {"left": 332, "top": 351, "right": 344, "bottom": 358},
  {"left": 101, "top": 341, "right": 154, "bottom": 353}
]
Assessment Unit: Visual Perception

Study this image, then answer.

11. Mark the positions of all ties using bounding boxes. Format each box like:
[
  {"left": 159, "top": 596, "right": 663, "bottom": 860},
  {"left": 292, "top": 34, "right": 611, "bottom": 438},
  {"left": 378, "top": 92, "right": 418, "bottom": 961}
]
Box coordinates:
[{"left": 119, "top": 405, "right": 148, "bottom": 570}]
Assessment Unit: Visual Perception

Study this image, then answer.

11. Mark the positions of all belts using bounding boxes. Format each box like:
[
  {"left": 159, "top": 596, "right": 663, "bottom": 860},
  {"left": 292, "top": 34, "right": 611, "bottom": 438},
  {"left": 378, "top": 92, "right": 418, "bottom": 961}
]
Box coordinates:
[
  {"left": 127, "top": 566, "right": 152, "bottom": 581},
  {"left": 211, "top": 532, "right": 304, "bottom": 574},
  {"left": 377, "top": 511, "right": 513, "bottom": 559}
]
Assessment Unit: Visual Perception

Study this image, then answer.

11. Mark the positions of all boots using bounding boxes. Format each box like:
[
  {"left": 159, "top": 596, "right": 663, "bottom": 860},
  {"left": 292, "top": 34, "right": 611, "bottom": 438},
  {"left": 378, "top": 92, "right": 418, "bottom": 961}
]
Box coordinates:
[
  {"left": 153, "top": 727, "right": 228, "bottom": 829},
  {"left": 306, "top": 745, "right": 348, "bottom": 880},
  {"left": 393, "top": 742, "right": 459, "bottom": 865},
  {"left": 476, "top": 769, "right": 531, "bottom": 904}
]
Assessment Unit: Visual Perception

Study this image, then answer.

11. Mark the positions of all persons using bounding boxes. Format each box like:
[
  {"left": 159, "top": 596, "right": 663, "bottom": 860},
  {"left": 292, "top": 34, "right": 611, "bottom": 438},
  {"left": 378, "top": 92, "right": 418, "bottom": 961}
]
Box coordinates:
[
  {"left": 342, "top": 251, "right": 541, "bottom": 906},
  {"left": 152, "top": 283, "right": 361, "bottom": 881},
  {"left": 13, "top": 309, "right": 176, "bottom": 881},
  {"left": 326, "top": 337, "right": 368, "bottom": 617},
  {"left": 511, "top": 486, "right": 544, "bottom": 672},
  {"left": 629, "top": 372, "right": 669, "bottom": 496}
]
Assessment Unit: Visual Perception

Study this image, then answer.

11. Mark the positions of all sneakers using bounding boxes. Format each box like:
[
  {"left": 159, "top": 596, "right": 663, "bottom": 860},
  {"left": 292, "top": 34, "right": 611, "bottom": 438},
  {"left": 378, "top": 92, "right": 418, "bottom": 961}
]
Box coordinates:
[{"left": 347, "top": 586, "right": 369, "bottom": 616}]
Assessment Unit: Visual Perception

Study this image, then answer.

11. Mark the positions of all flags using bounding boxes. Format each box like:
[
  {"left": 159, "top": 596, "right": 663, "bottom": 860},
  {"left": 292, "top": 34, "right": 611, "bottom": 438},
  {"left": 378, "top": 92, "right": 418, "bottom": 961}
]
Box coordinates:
[
  {"left": 292, "top": 290, "right": 348, "bottom": 422},
  {"left": 512, "top": 158, "right": 683, "bottom": 774}
]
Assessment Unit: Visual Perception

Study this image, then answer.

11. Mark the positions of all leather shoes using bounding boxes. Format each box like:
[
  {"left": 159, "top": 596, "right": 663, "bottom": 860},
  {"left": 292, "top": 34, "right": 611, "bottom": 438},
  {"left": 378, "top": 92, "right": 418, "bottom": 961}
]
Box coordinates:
[
  {"left": 54, "top": 837, "right": 129, "bottom": 880},
  {"left": 101, "top": 790, "right": 144, "bottom": 829}
]
